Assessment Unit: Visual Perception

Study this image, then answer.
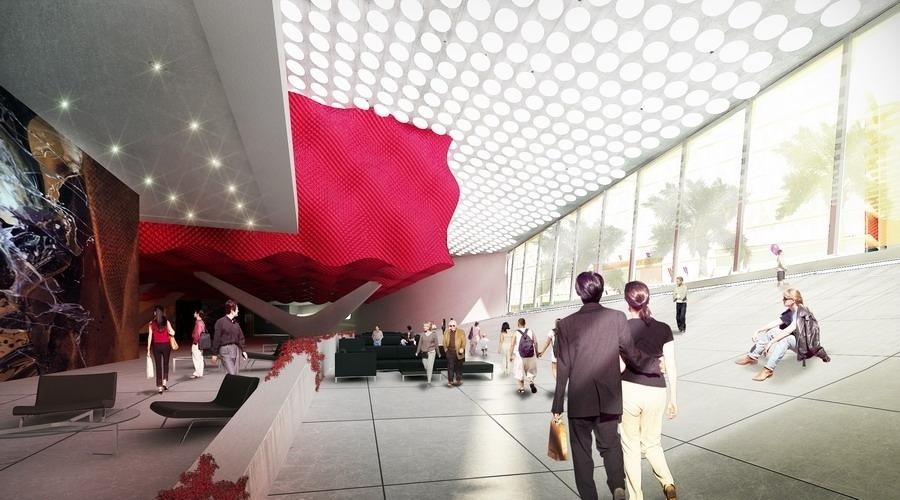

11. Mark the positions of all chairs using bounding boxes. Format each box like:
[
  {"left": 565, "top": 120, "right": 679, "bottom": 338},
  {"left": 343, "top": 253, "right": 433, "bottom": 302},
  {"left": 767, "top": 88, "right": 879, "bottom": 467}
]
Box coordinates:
[
  {"left": 245, "top": 344, "right": 281, "bottom": 369},
  {"left": 151, "top": 374, "right": 259, "bottom": 445}
]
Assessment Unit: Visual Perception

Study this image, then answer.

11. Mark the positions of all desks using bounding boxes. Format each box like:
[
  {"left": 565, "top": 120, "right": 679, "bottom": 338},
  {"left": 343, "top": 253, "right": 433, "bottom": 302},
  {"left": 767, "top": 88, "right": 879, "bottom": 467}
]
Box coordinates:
[
  {"left": 172, "top": 356, "right": 193, "bottom": 372},
  {"left": 0, "top": 407, "right": 140, "bottom": 456}
]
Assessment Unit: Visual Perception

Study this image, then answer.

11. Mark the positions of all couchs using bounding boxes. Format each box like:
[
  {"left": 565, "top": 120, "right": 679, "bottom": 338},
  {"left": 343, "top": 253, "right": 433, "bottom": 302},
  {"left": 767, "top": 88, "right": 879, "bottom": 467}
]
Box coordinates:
[{"left": 334, "top": 331, "right": 494, "bottom": 383}]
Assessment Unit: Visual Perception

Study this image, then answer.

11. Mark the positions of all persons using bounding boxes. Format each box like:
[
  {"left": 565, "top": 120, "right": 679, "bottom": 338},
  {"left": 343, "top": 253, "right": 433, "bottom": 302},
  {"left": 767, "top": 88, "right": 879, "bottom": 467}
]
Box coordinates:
[
  {"left": 735, "top": 287, "right": 831, "bottom": 381},
  {"left": 371, "top": 324, "right": 384, "bottom": 345},
  {"left": 400, "top": 317, "right": 561, "bottom": 394},
  {"left": 775, "top": 250, "right": 786, "bottom": 288},
  {"left": 189, "top": 309, "right": 209, "bottom": 380},
  {"left": 211, "top": 299, "right": 248, "bottom": 377},
  {"left": 550, "top": 270, "right": 668, "bottom": 500},
  {"left": 672, "top": 276, "right": 688, "bottom": 333},
  {"left": 146, "top": 305, "right": 175, "bottom": 393},
  {"left": 618, "top": 281, "right": 679, "bottom": 500}
]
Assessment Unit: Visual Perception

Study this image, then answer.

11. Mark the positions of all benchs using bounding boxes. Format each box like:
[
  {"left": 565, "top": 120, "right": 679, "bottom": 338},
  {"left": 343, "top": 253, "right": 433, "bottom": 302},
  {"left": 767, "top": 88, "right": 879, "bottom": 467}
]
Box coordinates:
[{"left": 13, "top": 372, "right": 117, "bottom": 429}]
